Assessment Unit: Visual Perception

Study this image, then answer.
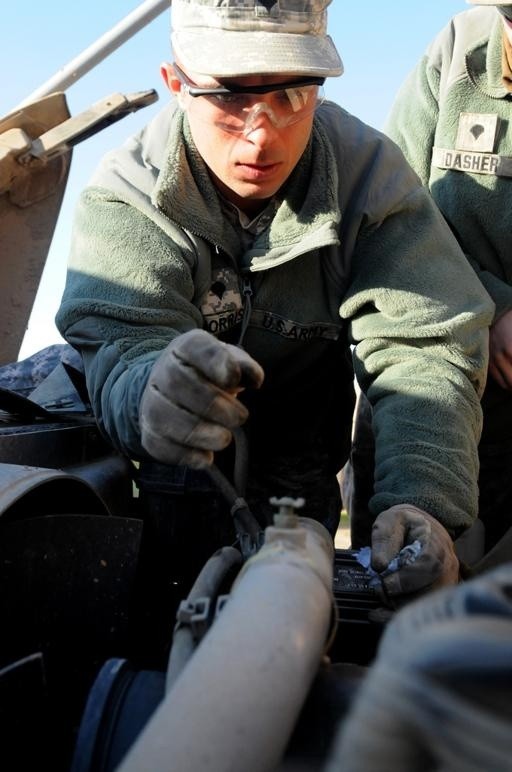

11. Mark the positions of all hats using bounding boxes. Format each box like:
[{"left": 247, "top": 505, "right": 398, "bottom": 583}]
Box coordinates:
[{"left": 171, "top": 1, "right": 345, "bottom": 78}]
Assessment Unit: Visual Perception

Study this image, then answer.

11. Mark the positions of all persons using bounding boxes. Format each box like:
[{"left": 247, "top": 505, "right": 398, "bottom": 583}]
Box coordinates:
[
  {"left": 54, "top": 0, "right": 497, "bottom": 628},
  {"left": 348, "top": 0, "right": 511, "bottom": 575}
]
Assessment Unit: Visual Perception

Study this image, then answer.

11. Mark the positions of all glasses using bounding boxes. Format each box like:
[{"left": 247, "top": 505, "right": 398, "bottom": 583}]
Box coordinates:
[{"left": 173, "top": 62, "right": 325, "bottom": 133}]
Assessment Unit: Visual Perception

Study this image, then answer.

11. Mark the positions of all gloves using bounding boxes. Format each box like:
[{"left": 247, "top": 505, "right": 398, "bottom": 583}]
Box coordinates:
[
  {"left": 138, "top": 328, "right": 265, "bottom": 471},
  {"left": 368, "top": 504, "right": 458, "bottom": 623}
]
